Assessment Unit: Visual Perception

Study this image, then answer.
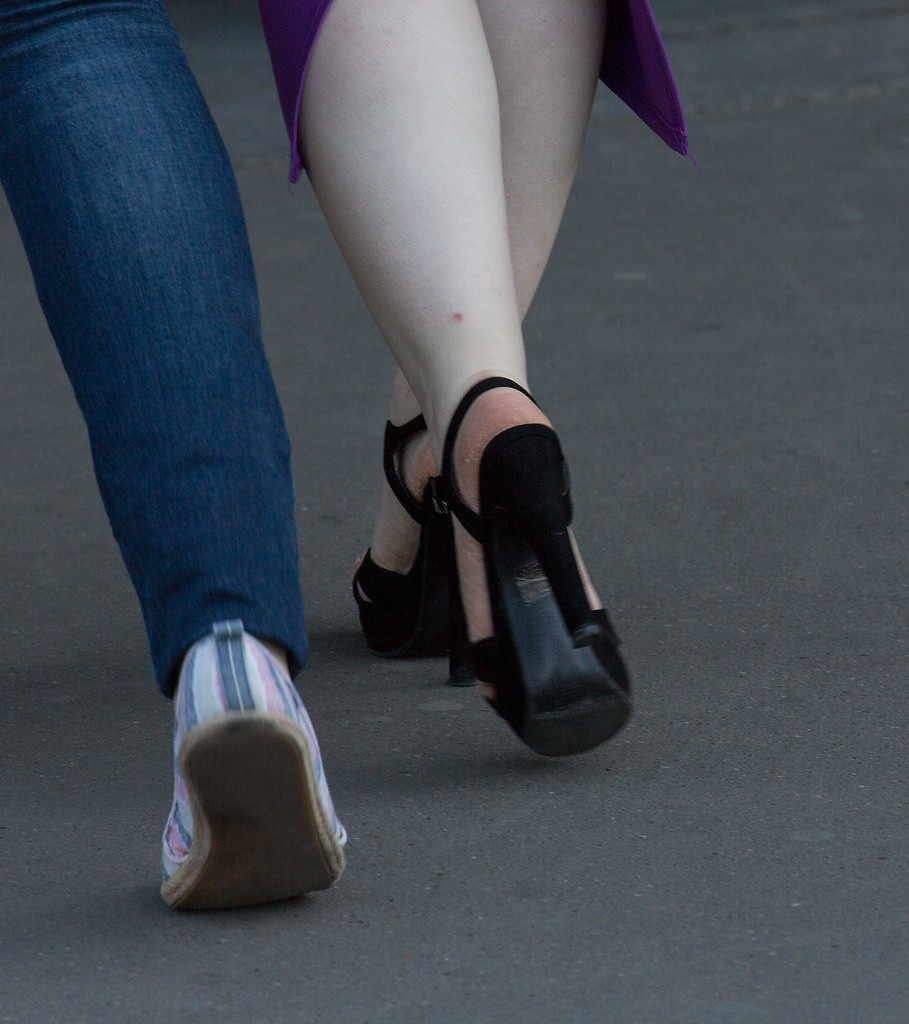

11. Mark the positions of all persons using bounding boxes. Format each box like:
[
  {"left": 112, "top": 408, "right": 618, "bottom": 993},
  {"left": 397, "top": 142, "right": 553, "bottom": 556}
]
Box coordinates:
[
  {"left": 0, "top": 0, "right": 348, "bottom": 912},
  {"left": 257, "top": 0, "right": 691, "bottom": 758}
]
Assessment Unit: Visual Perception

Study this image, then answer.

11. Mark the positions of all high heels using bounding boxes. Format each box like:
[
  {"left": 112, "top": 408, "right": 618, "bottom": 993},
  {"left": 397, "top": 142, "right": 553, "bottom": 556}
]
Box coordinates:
[
  {"left": 352, "top": 410, "right": 478, "bottom": 687},
  {"left": 450, "top": 376, "right": 634, "bottom": 754}
]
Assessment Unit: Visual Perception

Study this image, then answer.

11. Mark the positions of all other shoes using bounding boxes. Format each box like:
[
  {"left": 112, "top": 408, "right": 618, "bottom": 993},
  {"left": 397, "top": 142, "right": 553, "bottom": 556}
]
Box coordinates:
[{"left": 160, "top": 618, "right": 347, "bottom": 913}]
